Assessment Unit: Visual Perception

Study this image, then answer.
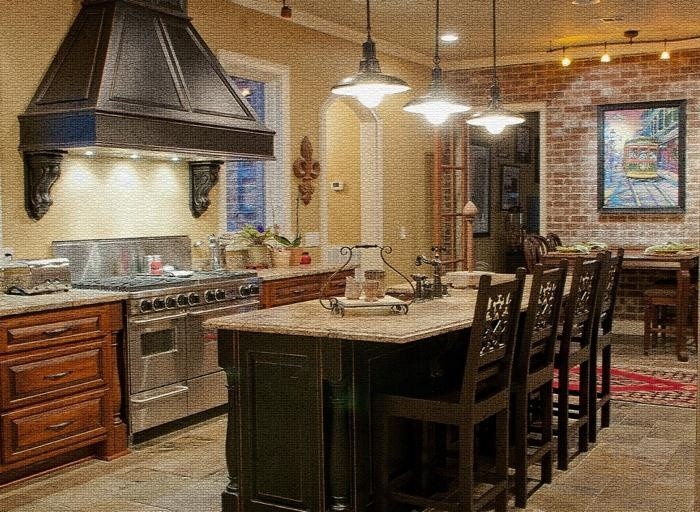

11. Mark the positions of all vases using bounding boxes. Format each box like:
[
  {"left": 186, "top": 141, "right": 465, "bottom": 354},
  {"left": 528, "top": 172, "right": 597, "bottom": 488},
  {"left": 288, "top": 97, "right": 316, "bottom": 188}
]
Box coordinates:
[
  {"left": 248, "top": 247, "right": 268, "bottom": 267},
  {"left": 226, "top": 245, "right": 247, "bottom": 267}
]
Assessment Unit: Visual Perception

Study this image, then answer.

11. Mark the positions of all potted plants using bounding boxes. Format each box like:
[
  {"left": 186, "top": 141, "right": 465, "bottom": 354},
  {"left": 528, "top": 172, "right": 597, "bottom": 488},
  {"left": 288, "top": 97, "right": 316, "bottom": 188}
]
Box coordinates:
[
  {"left": 275, "top": 234, "right": 303, "bottom": 265},
  {"left": 265, "top": 241, "right": 291, "bottom": 267}
]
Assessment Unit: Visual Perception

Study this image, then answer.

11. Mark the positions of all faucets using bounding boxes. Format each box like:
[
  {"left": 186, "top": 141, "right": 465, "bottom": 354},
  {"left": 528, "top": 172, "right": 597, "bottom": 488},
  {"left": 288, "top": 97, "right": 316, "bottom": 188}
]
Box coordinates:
[{"left": 414, "top": 254, "right": 444, "bottom": 298}]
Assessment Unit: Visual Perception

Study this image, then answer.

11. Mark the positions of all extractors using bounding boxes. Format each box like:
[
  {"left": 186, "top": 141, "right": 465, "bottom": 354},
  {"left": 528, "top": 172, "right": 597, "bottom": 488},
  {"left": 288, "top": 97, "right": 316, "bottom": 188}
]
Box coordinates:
[{"left": 18, "top": 1, "right": 277, "bottom": 162}]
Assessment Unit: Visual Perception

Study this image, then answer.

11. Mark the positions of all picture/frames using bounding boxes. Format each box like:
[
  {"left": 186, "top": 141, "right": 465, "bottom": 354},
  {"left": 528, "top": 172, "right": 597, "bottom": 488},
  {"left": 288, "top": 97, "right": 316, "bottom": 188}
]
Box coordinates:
[
  {"left": 596, "top": 99, "right": 688, "bottom": 216},
  {"left": 470, "top": 126, "right": 540, "bottom": 238}
]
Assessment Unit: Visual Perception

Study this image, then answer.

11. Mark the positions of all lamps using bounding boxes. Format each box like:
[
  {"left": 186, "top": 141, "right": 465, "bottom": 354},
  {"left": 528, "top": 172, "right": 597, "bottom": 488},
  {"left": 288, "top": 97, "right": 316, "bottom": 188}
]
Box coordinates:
[
  {"left": 402, "top": 0, "right": 472, "bottom": 125},
  {"left": 330, "top": 0, "right": 413, "bottom": 108},
  {"left": 546, "top": 30, "right": 700, "bottom": 68},
  {"left": 464, "top": 1, "right": 527, "bottom": 135}
]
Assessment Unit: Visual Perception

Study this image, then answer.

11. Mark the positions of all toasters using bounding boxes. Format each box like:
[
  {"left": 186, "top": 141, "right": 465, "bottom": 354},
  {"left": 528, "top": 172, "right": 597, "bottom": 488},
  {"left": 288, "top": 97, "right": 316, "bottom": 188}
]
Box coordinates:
[{"left": 0, "top": 256, "right": 72, "bottom": 296}]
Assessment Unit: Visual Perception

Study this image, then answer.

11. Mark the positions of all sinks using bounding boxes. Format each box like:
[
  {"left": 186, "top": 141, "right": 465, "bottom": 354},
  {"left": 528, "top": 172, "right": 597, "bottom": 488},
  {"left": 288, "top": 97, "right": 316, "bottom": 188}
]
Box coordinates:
[{"left": 386, "top": 287, "right": 433, "bottom": 303}]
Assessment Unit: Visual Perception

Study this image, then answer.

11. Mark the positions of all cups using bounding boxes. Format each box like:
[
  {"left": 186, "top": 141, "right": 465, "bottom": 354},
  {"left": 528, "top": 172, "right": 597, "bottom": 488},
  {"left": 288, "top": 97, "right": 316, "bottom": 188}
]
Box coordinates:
[{"left": 344, "top": 269, "right": 386, "bottom": 302}]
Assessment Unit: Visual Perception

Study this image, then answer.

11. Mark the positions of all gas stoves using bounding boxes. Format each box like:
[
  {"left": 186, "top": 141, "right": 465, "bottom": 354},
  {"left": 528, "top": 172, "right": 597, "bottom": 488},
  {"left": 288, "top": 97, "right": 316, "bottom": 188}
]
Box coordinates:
[{"left": 72, "top": 267, "right": 260, "bottom": 289}]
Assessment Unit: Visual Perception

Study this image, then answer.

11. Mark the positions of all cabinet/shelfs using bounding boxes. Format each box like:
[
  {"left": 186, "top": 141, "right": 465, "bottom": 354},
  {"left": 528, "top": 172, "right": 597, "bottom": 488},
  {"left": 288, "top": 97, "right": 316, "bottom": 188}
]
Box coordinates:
[
  {"left": 261, "top": 266, "right": 355, "bottom": 309},
  {"left": 0, "top": 288, "right": 132, "bottom": 490}
]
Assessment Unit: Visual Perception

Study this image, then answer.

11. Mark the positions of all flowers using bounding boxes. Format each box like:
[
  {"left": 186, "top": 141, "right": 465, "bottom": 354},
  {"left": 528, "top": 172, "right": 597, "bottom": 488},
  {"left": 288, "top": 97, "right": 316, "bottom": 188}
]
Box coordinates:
[{"left": 236, "top": 224, "right": 274, "bottom": 247}]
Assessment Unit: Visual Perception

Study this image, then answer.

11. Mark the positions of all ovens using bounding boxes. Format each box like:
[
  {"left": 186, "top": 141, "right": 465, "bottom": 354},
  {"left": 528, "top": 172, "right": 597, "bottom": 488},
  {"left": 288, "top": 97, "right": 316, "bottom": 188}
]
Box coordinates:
[{"left": 127, "top": 299, "right": 262, "bottom": 432}]
